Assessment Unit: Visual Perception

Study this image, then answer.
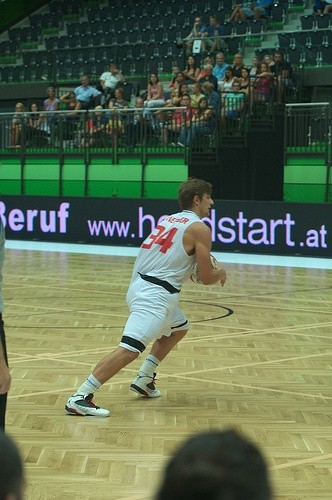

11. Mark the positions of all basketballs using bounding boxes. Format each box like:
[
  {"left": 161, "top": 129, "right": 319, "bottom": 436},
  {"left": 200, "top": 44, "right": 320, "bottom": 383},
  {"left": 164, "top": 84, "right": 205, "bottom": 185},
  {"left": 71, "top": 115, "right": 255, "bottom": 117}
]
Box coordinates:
[{"left": 190, "top": 253, "right": 219, "bottom": 285}]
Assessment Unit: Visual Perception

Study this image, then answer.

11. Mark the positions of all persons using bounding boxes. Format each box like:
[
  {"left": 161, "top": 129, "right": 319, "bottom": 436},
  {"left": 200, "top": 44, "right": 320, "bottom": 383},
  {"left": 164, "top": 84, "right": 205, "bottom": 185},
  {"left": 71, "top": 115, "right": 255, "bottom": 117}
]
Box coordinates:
[
  {"left": 65, "top": 180, "right": 227, "bottom": 417},
  {"left": 312, "top": 0, "right": 332, "bottom": 16},
  {"left": 185, "top": 14, "right": 228, "bottom": 56},
  {"left": 0, "top": 215, "right": 11, "bottom": 436},
  {"left": 224, "top": 0, "right": 289, "bottom": 24},
  {"left": 152, "top": 426, "right": 273, "bottom": 500},
  {"left": 0, "top": 433, "right": 25, "bottom": 500},
  {"left": 9, "top": 48, "right": 294, "bottom": 150}
]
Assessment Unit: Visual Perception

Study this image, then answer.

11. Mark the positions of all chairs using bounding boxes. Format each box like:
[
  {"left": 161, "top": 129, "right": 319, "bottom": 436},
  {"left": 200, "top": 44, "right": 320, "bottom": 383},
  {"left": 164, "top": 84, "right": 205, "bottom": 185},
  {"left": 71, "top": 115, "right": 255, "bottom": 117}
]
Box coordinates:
[{"left": 0, "top": 0, "right": 332, "bottom": 149}]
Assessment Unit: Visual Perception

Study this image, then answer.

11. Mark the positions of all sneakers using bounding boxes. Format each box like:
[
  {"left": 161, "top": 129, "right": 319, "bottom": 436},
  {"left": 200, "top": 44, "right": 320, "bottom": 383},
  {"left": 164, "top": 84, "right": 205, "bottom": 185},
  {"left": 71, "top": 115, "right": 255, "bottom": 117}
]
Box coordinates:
[
  {"left": 65, "top": 392, "right": 109, "bottom": 417},
  {"left": 129, "top": 373, "right": 160, "bottom": 398}
]
